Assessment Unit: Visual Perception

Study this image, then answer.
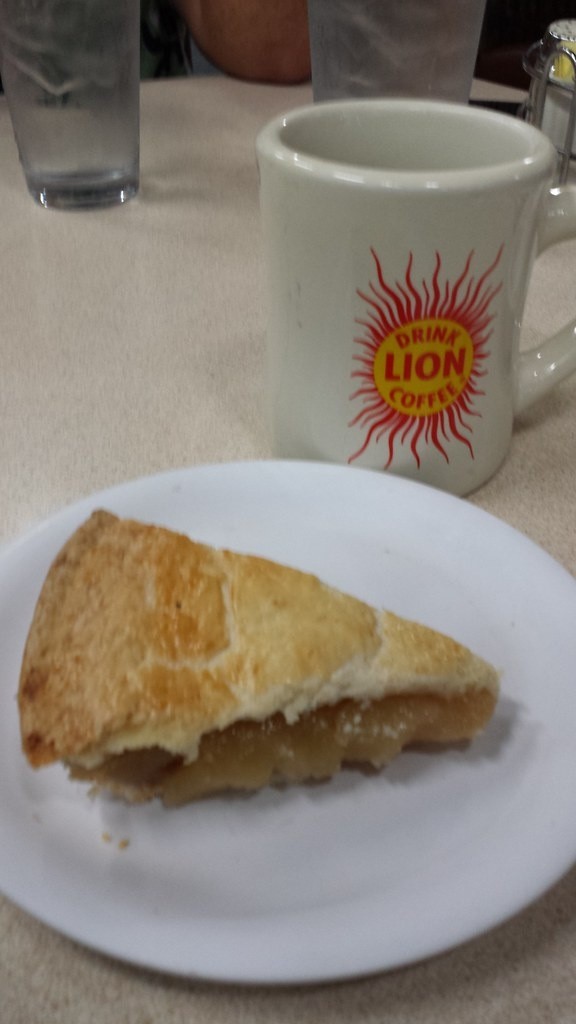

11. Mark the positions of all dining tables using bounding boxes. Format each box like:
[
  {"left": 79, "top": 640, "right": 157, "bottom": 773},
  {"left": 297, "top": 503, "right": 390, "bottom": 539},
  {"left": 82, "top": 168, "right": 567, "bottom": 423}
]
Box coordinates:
[{"left": 0, "top": 78, "right": 576, "bottom": 1024}]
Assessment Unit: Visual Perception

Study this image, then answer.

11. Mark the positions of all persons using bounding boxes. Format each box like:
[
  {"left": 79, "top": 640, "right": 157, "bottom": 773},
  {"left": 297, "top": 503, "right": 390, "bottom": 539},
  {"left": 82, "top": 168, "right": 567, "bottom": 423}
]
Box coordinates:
[{"left": 188, "top": 0, "right": 576, "bottom": 119}]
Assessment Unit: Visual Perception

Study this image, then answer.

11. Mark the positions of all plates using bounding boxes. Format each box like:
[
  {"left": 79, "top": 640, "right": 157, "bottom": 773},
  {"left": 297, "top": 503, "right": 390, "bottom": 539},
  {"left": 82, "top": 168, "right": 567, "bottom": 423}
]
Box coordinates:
[{"left": 0, "top": 459, "right": 576, "bottom": 990}]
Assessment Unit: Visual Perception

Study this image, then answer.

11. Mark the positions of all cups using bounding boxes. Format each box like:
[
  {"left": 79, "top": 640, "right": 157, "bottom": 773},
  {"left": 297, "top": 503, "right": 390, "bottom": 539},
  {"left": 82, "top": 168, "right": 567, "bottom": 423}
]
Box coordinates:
[
  {"left": 306, "top": 0, "right": 485, "bottom": 131},
  {"left": 1, "top": 0, "right": 144, "bottom": 211},
  {"left": 255, "top": 102, "right": 576, "bottom": 499}
]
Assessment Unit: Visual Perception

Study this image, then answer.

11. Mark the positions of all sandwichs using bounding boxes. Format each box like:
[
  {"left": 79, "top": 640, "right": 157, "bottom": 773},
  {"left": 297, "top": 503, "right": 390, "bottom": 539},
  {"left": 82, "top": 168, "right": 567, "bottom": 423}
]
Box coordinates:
[{"left": 18, "top": 509, "right": 502, "bottom": 805}]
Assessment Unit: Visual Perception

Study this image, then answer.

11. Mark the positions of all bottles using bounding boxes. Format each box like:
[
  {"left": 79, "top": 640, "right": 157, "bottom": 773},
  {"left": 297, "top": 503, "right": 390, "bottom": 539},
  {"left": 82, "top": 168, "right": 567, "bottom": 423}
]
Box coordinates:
[{"left": 530, "top": 19, "right": 576, "bottom": 158}]
